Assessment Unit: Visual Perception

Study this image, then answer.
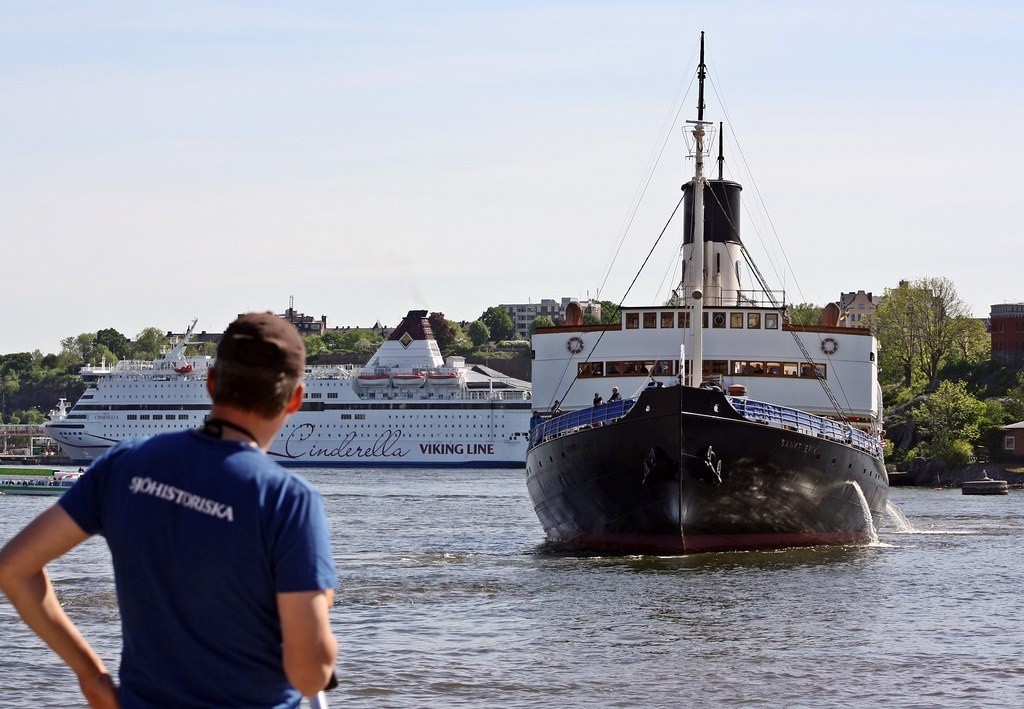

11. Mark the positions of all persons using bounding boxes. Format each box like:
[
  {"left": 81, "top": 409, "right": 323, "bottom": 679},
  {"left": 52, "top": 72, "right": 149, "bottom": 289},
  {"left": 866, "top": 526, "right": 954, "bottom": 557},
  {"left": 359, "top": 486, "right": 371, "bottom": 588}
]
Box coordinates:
[
  {"left": 530, "top": 411, "right": 545, "bottom": 441},
  {"left": 0, "top": 311, "right": 338, "bottom": 709},
  {"left": 607, "top": 387, "right": 622, "bottom": 403},
  {"left": 580, "top": 363, "right": 809, "bottom": 378},
  {"left": 594, "top": 393, "right": 603, "bottom": 407},
  {"left": 551, "top": 400, "right": 562, "bottom": 417},
  {"left": 881, "top": 430, "right": 886, "bottom": 440}
]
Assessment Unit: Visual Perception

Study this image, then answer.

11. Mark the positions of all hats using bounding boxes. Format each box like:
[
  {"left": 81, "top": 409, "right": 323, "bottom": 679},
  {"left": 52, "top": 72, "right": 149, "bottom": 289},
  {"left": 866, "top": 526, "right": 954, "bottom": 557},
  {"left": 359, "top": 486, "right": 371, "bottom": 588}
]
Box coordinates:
[{"left": 217, "top": 312, "right": 306, "bottom": 378}]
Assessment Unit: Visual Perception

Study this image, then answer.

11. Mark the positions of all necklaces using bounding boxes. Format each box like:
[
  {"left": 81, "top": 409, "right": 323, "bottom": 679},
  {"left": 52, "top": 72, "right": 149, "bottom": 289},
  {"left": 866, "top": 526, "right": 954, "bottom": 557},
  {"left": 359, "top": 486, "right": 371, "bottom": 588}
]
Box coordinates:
[{"left": 204, "top": 418, "right": 260, "bottom": 444}]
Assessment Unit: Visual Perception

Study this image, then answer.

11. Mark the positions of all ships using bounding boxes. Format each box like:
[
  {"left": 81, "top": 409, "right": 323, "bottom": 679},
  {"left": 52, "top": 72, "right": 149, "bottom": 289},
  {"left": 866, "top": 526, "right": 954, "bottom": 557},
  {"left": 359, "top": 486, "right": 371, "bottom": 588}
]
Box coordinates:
[{"left": 39, "top": 307, "right": 533, "bottom": 469}]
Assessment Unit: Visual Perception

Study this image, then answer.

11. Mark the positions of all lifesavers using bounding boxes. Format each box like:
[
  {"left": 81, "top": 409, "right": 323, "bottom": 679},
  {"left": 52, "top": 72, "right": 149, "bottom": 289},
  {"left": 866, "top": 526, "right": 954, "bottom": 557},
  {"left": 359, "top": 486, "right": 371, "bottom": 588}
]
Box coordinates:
[
  {"left": 567, "top": 337, "right": 583, "bottom": 353},
  {"left": 821, "top": 337, "right": 838, "bottom": 355}
]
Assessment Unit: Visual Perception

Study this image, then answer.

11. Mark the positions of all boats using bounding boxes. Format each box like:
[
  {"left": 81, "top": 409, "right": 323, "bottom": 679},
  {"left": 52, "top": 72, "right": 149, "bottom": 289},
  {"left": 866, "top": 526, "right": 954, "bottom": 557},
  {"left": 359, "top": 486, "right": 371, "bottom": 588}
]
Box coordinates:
[
  {"left": 357, "top": 373, "right": 389, "bottom": 386},
  {"left": 526, "top": 27, "right": 892, "bottom": 552},
  {"left": 391, "top": 373, "right": 425, "bottom": 386},
  {"left": 429, "top": 373, "right": 461, "bottom": 386},
  {"left": 0, "top": 464, "right": 86, "bottom": 496}
]
